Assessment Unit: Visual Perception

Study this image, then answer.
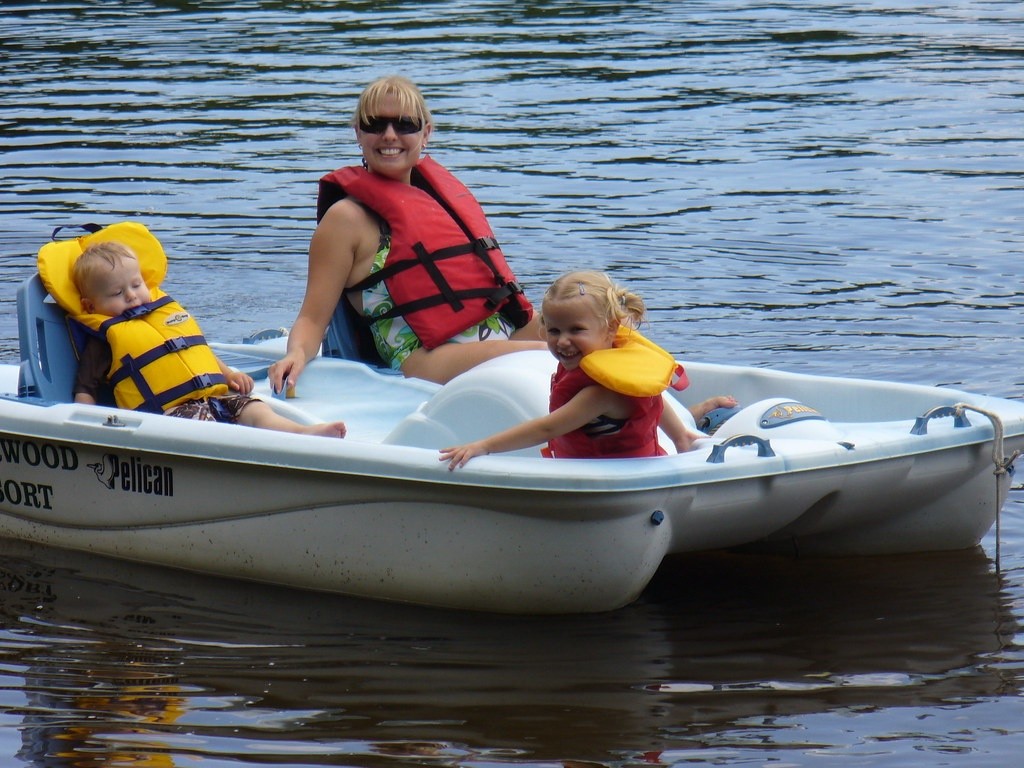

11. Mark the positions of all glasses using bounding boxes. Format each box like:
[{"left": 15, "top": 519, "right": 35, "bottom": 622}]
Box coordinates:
[{"left": 359, "top": 115, "right": 426, "bottom": 135}]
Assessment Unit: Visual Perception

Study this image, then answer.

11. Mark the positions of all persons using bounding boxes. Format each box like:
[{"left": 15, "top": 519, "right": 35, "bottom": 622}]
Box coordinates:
[
  {"left": 439, "top": 271, "right": 711, "bottom": 471},
  {"left": 72, "top": 243, "right": 346, "bottom": 439},
  {"left": 268, "top": 76, "right": 738, "bottom": 424}
]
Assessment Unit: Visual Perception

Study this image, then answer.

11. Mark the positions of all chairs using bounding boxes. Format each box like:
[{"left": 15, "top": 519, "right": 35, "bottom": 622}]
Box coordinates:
[
  {"left": 322, "top": 290, "right": 385, "bottom": 369},
  {"left": 17, "top": 273, "right": 98, "bottom": 403}
]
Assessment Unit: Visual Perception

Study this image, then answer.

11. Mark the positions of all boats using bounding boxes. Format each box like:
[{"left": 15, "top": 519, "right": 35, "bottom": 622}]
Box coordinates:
[{"left": 0, "top": 330, "right": 1024, "bottom": 618}]
